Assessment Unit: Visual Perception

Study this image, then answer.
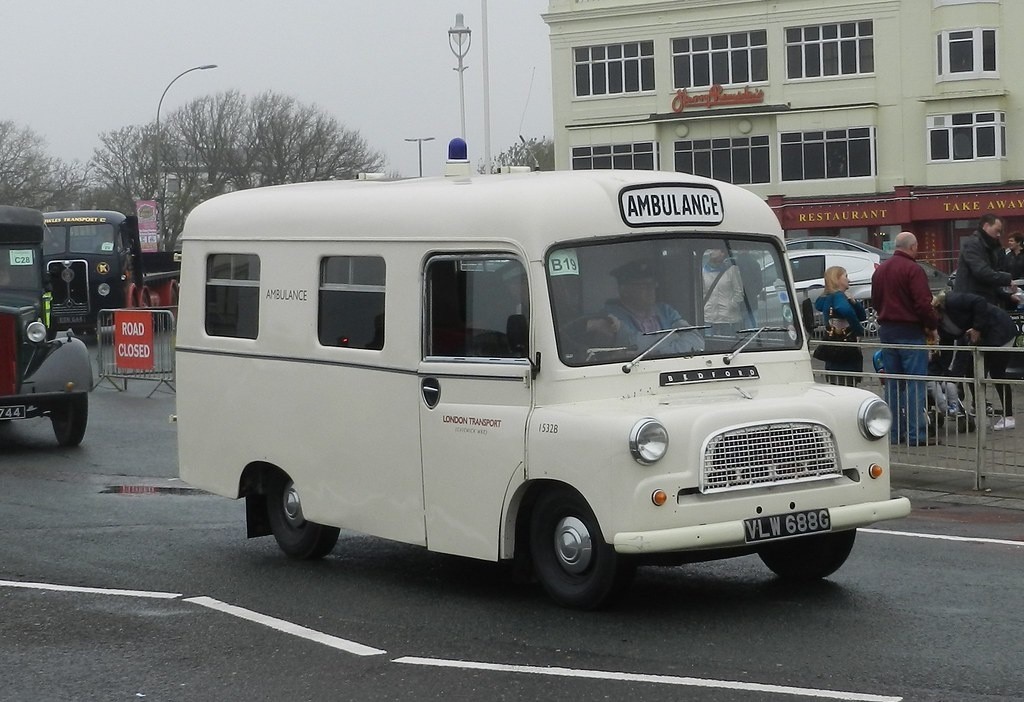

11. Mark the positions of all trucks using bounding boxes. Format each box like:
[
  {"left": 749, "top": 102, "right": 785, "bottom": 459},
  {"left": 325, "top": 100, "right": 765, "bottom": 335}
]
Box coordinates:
[{"left": 43, "top": 209, "right": 181, "bottom": 340}]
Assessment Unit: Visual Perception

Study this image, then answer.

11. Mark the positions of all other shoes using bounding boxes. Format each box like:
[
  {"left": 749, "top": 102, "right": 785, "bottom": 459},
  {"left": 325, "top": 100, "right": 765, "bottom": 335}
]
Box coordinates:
[
  {"left": 944, "top": 402, "right": 962, "bottom": 415},
  {"left": 906, "top": 439, "right": 941, "bottom": 446}
]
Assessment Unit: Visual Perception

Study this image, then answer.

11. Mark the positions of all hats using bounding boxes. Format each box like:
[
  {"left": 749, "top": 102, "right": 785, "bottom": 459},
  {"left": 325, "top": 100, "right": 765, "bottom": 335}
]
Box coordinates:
[{"left": 609, "top": 258, "right": 657, "bottom": 279}]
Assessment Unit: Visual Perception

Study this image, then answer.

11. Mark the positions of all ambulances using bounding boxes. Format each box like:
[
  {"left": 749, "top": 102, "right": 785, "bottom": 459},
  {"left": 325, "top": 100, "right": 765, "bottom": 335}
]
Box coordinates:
[{"left": 174, "top": 138, "right": 912, "bottom": 610}]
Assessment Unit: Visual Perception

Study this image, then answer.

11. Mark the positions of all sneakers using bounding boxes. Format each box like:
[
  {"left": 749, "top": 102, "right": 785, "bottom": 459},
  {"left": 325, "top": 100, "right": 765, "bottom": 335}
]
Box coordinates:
[
  {"left": 994, "top": 416, "right": 1016, "bottom": 430},
  {"left": 970, "top": 402, "right": 1004, "bottom": 416}
]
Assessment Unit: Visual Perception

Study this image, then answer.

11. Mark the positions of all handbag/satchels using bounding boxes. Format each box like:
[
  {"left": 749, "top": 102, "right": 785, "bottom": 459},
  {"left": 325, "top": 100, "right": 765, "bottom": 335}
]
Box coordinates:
[{"left": 829, "top": 307, "right": 851, "bottom": 337}]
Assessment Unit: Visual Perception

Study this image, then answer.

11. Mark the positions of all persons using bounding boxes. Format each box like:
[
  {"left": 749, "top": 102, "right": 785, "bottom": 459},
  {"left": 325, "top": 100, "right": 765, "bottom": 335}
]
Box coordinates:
[
  {"left": 812, "top": 266, "right": 866, "bottom": 387},
  {"left": 928, "top": 213, "right": 1024, "bottom": 432},
  {"left": 871, "top": 231, "right": 942, "bottom": 447},
  {"left": 493, "top": 248, "right": 765, "bottom": 353}
]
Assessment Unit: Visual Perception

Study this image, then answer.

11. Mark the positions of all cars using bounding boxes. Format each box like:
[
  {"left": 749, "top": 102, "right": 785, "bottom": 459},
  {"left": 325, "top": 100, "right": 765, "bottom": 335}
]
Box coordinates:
[
  {"left": 753, "top": 237, "right": 952, "bottom": 338},
  {"left": 0, "top": 203, "right": 94, "bottom": 451}
]
